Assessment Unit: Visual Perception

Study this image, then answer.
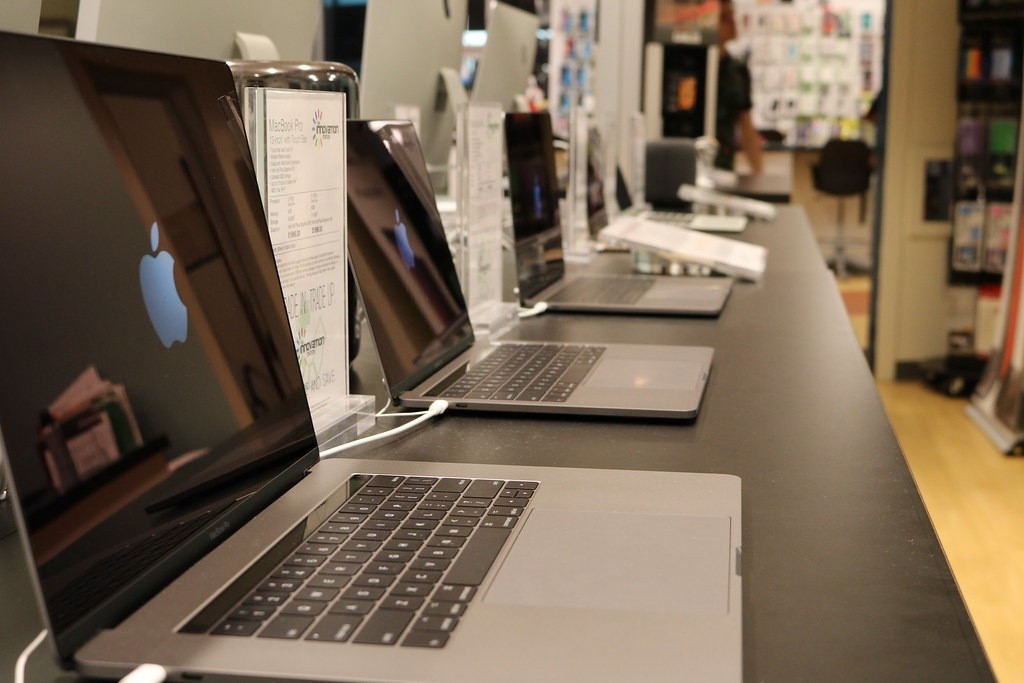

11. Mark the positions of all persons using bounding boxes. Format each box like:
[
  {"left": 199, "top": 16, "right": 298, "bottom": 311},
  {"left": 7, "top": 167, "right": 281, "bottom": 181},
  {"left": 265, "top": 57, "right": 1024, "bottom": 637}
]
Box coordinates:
[{"left": 715, "top": 11, "right": 762, "bottom": 173}]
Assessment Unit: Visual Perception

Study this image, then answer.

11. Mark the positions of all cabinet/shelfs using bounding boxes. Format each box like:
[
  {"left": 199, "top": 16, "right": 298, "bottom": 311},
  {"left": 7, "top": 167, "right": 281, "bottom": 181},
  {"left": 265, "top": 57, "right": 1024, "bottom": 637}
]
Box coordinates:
[{"left": 948, "top": 0, "right": 1024, "bottom": 286}]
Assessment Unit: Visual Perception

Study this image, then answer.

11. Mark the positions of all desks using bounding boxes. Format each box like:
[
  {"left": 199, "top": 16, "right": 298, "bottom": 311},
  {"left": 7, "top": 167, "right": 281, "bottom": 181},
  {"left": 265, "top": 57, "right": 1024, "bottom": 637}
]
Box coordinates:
[{"left": 0, "top": 203, "right": 1001, "bottom": 683}]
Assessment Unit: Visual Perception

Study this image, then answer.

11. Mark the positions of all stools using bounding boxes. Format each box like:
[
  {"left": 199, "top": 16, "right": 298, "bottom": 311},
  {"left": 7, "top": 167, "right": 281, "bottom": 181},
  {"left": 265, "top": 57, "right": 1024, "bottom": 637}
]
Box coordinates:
[{"left": 811, "top": 134, "right": 874, "bottom": 276}]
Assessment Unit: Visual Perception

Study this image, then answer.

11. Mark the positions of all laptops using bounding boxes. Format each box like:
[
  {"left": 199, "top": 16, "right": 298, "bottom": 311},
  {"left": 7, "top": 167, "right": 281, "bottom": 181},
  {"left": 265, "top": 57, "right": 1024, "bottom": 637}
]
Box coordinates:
[{"left": 0, "top": 28, "right": 749, "bottom": 683}]
